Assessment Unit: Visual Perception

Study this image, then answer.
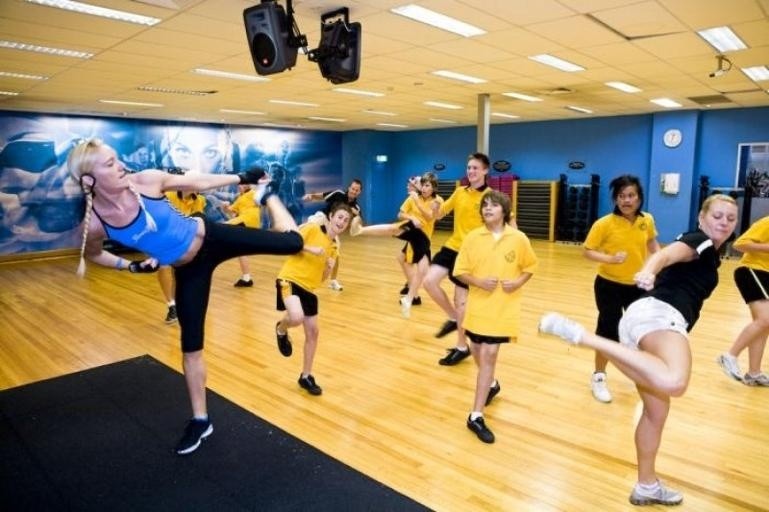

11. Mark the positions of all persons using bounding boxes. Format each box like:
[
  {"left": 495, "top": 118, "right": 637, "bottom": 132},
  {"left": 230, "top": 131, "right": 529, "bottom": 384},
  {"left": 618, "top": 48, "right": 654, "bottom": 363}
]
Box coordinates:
[
  {"left": 454, "top": 189, "right": 538, "bottom": 442},
  {"left": 224, "top": 172, "right": 270, "bottom": 288},
  {"left": 423, "top": 153, "right": 491, "bottom": 365},
  {"left": 542, "top": 193, "right": 737, "bottom": 504},
  {"left": 398, "top": 177, "right": 426, "bottom": 294},
  {"left": 275, "top": 202, "right": 353, "bottom": 396},
  {"left": 350, "top": 171, "right": 441, "bottom": 317},
  {"left": 301, "top": 181, "right": 363, "bottom": 293},
  {"left": 720, "top": 215, "right": 768, "bottom": 390},
  {"left": 158, "top": 165, "right": 207, "bottom": 324},
  {"left": 68, "top": 141, "right": 304, "bottom": 454},
  {"left": 581, "top": 175, "right": 660, "bottom": 403}
]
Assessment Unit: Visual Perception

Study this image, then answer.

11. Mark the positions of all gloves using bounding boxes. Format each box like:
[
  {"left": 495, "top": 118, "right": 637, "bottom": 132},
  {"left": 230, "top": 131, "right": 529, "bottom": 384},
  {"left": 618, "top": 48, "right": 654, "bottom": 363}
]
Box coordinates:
[
  {"left": 237, "top": 167, "right": 265, "bottom": 184},
  {"left": 129, "top": 258, "right": 159, "bottom": 272}
]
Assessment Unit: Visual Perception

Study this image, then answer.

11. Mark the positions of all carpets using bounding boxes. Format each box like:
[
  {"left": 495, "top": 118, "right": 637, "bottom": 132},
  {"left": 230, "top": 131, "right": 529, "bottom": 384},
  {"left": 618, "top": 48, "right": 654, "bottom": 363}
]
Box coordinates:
[{"left": 0, "top": 354, "right": 435, "bottom": 512}]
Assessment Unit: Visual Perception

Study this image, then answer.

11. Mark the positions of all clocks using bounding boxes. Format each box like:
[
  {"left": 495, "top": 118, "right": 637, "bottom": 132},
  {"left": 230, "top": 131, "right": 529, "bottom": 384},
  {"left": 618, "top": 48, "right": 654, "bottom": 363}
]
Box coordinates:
[{"left": 663, "top": 129, "right": 682, "bottom": 147}]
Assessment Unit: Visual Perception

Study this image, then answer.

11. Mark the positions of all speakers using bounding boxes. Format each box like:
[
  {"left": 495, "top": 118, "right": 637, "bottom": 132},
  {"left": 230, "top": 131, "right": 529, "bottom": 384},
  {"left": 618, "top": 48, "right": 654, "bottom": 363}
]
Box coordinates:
[
  {"left": 243, "top": 1, "right": 298, "bottom": 76},
  {"left": 309, "top": 7, "right": 361, "bottom": 85}
]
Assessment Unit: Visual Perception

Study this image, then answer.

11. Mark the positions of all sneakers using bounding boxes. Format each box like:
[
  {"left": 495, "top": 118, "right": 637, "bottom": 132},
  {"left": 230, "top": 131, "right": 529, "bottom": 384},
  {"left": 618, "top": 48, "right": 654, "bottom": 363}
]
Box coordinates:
[
  {"left": 177, "top": 415, "right": 213, "bottom": 454},
  {"left": 399, "top": 296, "right": 421, "bottom": 304},
  {"left": 485, "top": 380, "right": 500, "bottom": 406},
  {"left": 592, "top": 372, "right": 611, "bottom": 402},
  {"left": 165, "top": 305, "right": 179, "bottom": 323},
  {"left": 298, "top": 372, "right": 322, "bottom": 395},
  {"left": 399, "top": 298, "right": 411, "bottom": 320},
  {"left": 276, "top": 321, "right": 292, "bottom": 356},
  {"left": 256, "top": 181, "right": 280, "bottom": 205},
  {"left": 743, "top": 372, "right": 768, "bottom": 386},
  {"left": 328, "top": 280, "right": 343, "bottom": 290},
  {"left": 538, "top": 312, "right": 585, "bottom": 346},
  {"left": 439, "top": 348, "right": 471, "bottom": 365},
  {"left": 401, "top": 284, "right": 409, "bottom": 294},
  {"left": 234, "top": 279, "right": 253, "bottom": 287},
  {"left": 467, "top": 413, "right": 494, "bottom": 443},
  {"left": 716, "top": 352, "right": 745, "bottom": 381},
  {"left": 629, "top": 481, "right": 683, "bottom": 505},
  {"left": 436, "top": 320, "right": 457, "bottom": 338},
  {"left": 349, "top": 215, "right": 361, "bottom": 237}
]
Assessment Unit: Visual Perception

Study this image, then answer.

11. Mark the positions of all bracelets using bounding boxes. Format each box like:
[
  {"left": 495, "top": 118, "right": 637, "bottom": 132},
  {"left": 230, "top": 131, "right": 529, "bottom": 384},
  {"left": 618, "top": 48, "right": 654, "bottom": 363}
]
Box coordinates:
[
  {"left": 115, "top": 257, "right": 123, "bottom": 270},
  {"left": 231, "top": 175, "right": 238, "bottom": 185}
]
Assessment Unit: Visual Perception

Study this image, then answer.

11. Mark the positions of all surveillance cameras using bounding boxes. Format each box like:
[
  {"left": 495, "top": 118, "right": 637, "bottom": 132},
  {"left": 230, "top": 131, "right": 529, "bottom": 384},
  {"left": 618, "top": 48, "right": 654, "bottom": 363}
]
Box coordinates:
[{"left": 709, "top": 55, "right": 731, "bottom": 77}]
